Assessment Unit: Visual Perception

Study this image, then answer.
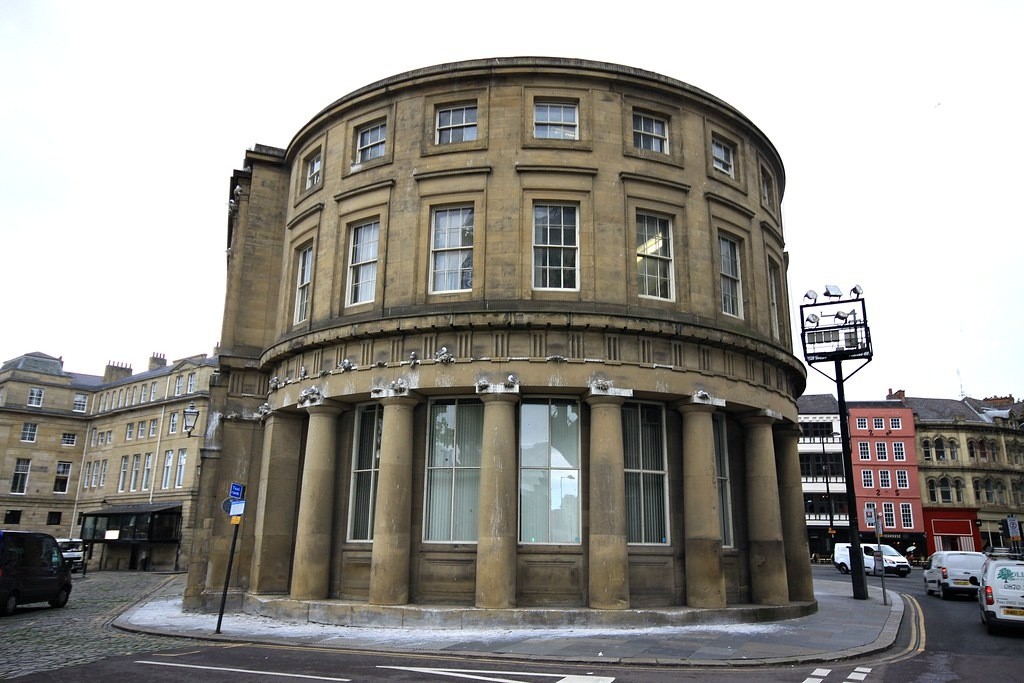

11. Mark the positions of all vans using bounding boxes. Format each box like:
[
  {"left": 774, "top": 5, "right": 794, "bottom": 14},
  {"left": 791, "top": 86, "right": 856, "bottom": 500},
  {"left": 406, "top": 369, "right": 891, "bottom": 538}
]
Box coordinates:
[
  {"left": 833, "top": 542, "right": 912, "bottom": 578},
  {"left": 923, "top": 550, "right": 987, "bottom": 600},
  {"left": 969, "top": 557, "right": 1024, "bottom": 635},
  {"left": 0, "top": 529, "right": 73, "bottom": 616},
  {"left": 55, "top": 538, "right": 86, "bottom": 570}
]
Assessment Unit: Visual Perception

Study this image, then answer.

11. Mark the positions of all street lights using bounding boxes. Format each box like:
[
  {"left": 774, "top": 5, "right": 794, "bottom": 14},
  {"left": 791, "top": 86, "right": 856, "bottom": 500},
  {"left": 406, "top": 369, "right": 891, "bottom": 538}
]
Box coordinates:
[
  {"left": 799, "top": 284, "right": 873, "bottom": 600},
  {"left": 819, "top": 432, "right": 840, "bottom": 554}
]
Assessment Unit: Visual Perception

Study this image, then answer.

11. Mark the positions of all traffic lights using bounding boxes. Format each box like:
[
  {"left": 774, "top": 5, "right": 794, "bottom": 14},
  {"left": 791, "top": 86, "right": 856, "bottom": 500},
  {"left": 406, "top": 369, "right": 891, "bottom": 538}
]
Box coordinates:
[{"left": 998, "top": 519, "right": 1010, "bottom": 538}]
